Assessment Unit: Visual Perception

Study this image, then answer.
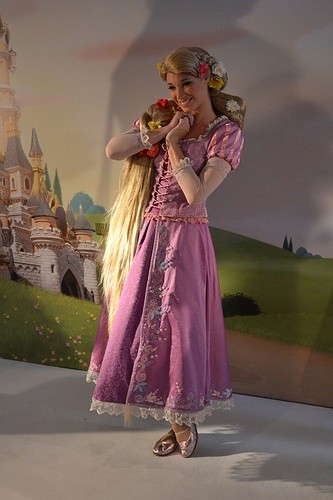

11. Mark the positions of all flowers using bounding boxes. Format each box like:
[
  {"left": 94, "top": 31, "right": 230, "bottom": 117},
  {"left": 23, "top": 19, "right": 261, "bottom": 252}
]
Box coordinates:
[
  {"left": 226, "top": 99, "right": 241, "bottom": 113},
  {"left": 156, "top": 98, "right": 169, "bottom": 111},
  {"left": 147, "top": 119, "right": 162, "bottom": 130},
  {"left": 146, "top": 143, "right": 162, "bottom": 159},
  {"left": 196, "top": 62, "right": 228, "bottom": 91}
]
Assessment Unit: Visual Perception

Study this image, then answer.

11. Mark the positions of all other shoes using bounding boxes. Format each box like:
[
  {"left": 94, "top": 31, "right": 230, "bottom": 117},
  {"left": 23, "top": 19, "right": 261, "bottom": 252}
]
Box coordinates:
[
  {"left": 175, "top": 423, "right": 198, "bottom": 458},
  {"left": 152, "top": 429, "right": 178, "bottom": 455}
]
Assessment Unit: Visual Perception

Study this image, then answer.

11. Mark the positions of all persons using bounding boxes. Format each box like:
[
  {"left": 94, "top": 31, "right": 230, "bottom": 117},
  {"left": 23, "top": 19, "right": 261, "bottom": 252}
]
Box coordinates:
[{"left": 86, "top": 47, "right": 247, "bottom": 458}]
